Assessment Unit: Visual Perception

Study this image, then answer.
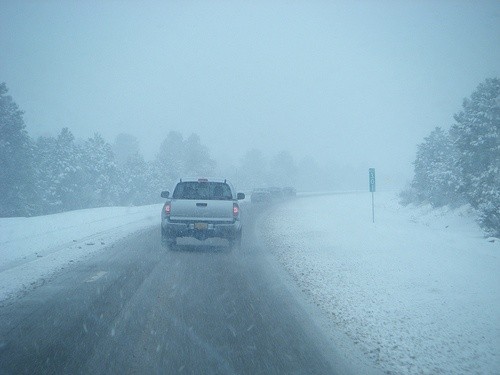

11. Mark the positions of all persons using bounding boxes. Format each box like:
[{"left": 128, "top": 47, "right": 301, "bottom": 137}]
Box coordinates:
[{"left": 212, "top": 184, "right": 224, "bottom": 197}]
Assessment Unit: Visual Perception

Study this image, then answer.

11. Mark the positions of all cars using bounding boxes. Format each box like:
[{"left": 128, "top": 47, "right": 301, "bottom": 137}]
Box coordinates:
[{"left": 160, "top": 175, "right": 245, "bottom": 247}]
